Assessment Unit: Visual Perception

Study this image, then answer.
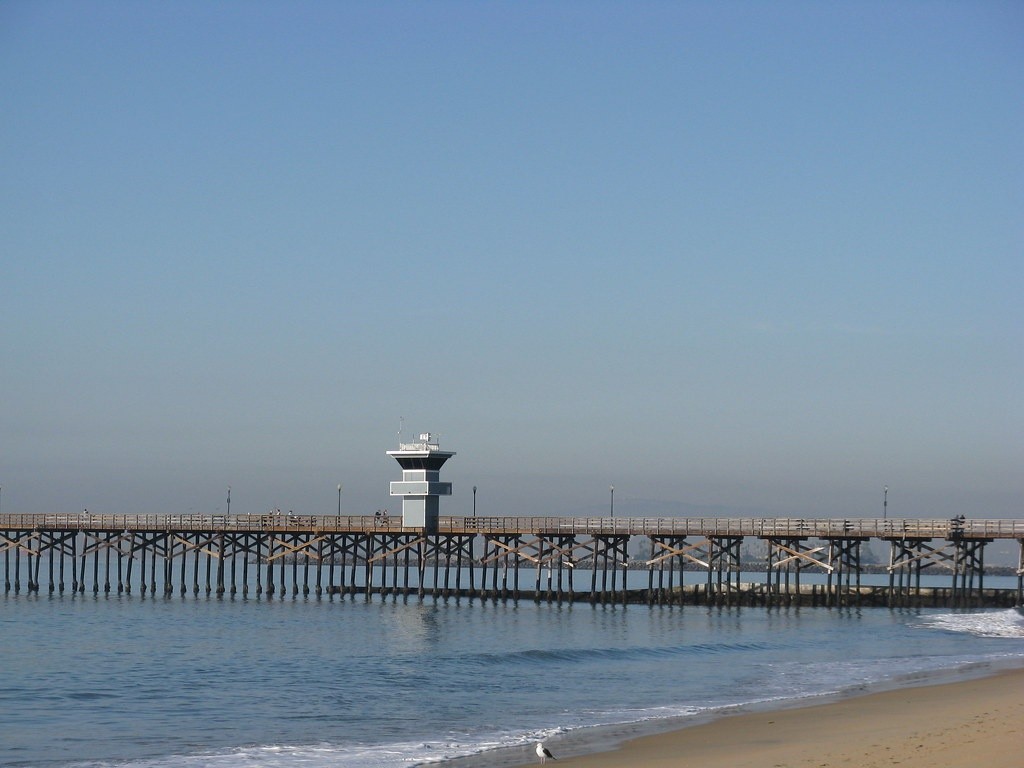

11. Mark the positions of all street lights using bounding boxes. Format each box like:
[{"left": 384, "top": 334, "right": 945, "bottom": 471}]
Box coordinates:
[
  {"left": 338, "top": 484, "right": 341, "bottom": 527},
  {"left": 472, "top": 486, "right": 478, "bottom": 528},
  {"left": 227, "top": 487, "right": 231, "bottom": 525},
  {"left": 883, "top": 485, "right": 888, "bottom": 530},
  {"left": 610, "top": 482, "right": 615, "bottom": 527}
]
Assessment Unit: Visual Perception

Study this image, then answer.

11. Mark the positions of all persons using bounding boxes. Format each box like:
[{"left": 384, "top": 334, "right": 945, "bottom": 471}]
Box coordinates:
[
  {"left": 375, "top": 509, "right": 390, "bottom": 527},
  {"left": 84, "top": 509, "right": 89, "bottom": 525},
  {"left": 954, "top": 515, "right": 965, "bottom": 533},
  {"left": 260, "top": 509, "right": 296, "bottom": 525}
]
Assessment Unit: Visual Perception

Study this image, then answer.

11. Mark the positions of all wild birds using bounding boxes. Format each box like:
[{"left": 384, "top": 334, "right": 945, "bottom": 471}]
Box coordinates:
[{"left": 536, "top": 743, "right": 557, "bottom": 766}]
